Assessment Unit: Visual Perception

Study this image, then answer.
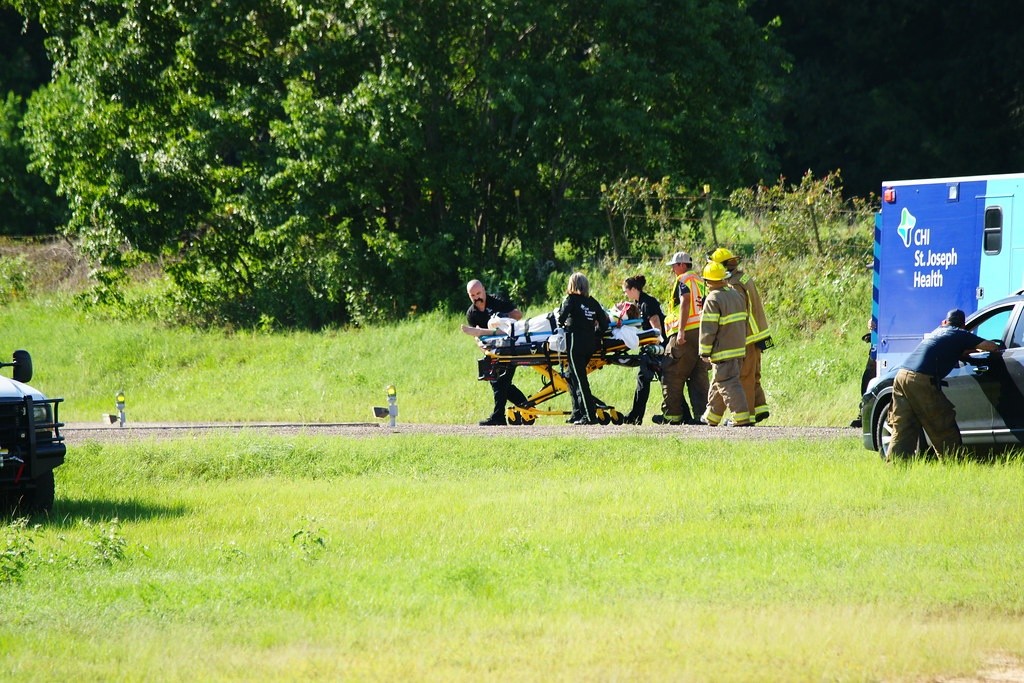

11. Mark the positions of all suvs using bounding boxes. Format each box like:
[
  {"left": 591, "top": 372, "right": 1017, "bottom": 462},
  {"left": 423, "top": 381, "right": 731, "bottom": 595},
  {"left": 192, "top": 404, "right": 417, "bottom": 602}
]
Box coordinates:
[
  {"left": 863, "top": 288, "right": 1024, "bottom": 461},
  {"left": 0, "top": 350, "right": 67, "bottom": 518}
]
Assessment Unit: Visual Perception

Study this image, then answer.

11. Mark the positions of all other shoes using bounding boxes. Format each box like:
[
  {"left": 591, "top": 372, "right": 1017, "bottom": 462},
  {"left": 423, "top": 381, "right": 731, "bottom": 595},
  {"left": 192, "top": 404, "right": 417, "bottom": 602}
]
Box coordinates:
[
  {"left": 727, "top": 420, "right": 751, "bottom": 426},
  {"left": 756, "top": 412, "right": 769, "bottom": 423},
  {"left": 479, "top": 415, "right": 507, "bottom": 425},
  {"left": 650, "top": 414, "right": 681, "bottom": 425},
  {"left": 622, "top": 411, "right": 642, "bottom": 425},
  {"left": 566, "top": 416, "right": 579, "bottom": 422},
  {"left": 699, "top": 416, "right": 717, "bottom": 427},
  {"left": 574, "top": 418, "right": 594, "bottom": 426}
]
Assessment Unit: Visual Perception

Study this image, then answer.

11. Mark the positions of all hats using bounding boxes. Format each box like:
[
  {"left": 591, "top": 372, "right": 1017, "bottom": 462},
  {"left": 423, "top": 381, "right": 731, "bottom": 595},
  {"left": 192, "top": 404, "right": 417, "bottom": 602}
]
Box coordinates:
[
  {"left": 666, "top": 252, "right": 692, "bottom": 266},
  {"left": 947, "top": 309, "right": 965, "bottom": 324}
]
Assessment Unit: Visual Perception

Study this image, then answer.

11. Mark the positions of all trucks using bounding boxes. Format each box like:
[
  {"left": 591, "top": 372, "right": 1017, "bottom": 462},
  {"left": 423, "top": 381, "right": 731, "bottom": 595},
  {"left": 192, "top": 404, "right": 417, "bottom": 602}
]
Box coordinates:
[{"left": 870, "top": 171, "right": 1024, "bottom": 375}]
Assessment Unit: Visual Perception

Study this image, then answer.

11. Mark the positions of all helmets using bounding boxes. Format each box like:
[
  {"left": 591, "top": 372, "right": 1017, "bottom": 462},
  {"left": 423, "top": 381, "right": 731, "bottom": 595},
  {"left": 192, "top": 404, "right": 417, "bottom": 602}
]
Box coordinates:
[
  {"left": 710, "top": 248, "right": 734, "bottom": 263},
  {"left": 701, "top": 261, "right": 726, "bottom": 281}
]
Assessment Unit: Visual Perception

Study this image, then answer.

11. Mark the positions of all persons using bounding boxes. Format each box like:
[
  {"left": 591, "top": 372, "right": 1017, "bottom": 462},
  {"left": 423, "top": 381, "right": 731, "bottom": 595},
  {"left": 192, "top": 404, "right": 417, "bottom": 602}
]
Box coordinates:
[
  {"left": 850, "top": 318, "right": 876, "bottom": 428},
  {"left": 465, "top": 279, "right": 528, "bottom": 425},
  {"left": 885, "top": 309, "right": 998, "bottom": 463},
  {"left": 689, "top": 261, "right": 750, "bottom": 428},
  {"left": 621, "top": 274, "right": 693, "bottom": 426},
  {"left": 461, "top": 301, "right": 640, "bottom": 338},
  {"left": 707, "top": 247, "right": 775, "bottom": 425},
  {"left": 557, "top": 273, "right": 609, "bottom": 425},
  {"left": 651, "top": 252, "right": 712, "bottom": 425}
]
